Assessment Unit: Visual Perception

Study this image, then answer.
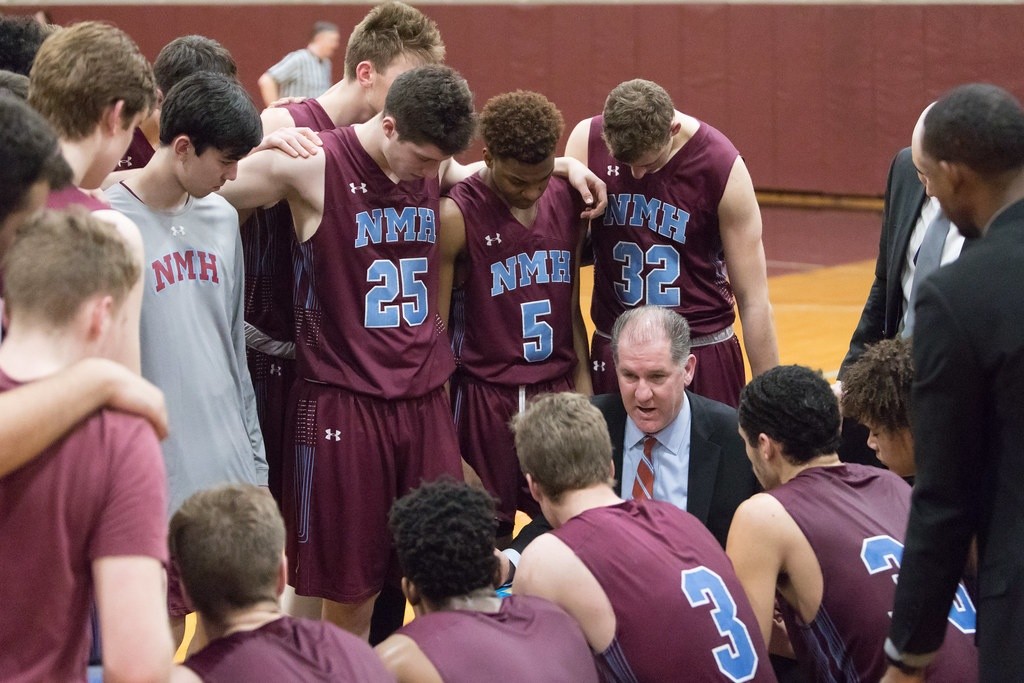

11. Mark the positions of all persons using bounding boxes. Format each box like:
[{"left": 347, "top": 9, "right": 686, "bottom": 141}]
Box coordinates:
[
  {"left": 877, "top": 81, "right": 1024, "bottom": 683},
  {"left": 0, "top": 1, "right": 975, "bottom": 683}
]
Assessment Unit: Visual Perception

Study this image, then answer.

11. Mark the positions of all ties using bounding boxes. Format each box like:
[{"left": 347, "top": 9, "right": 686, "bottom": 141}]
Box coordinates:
[
  {"left": 900, "top": 207, "right": 952, "bottom": 339},
  {"left": 631, "top": 434, "right": 656, "bottom": 500}
]
